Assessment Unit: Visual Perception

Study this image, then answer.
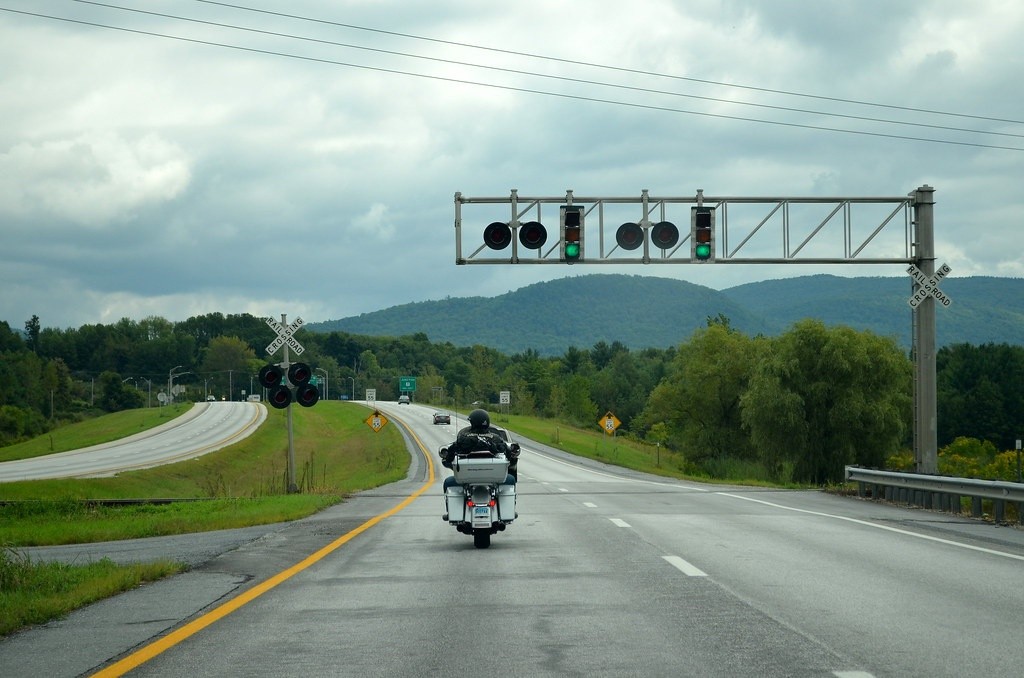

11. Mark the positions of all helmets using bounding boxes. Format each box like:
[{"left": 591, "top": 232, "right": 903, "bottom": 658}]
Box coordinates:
[{"left": 467, "top": 409, "right": 490, "bottom": 433}]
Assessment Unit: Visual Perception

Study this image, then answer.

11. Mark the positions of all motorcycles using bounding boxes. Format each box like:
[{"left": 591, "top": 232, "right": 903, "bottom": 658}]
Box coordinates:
[{"left": 438, "top": 425, "right": 521, "bottom": 548}]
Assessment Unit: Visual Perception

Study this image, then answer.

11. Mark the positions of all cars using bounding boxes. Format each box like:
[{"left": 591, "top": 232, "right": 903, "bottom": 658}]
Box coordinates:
[
  {"left": 433, "top": 412, "right": 450, "bottom": 424},
  {"left": 398, "top": 395, "right": 410, "bottom": 405},
  {"left": 207, "top": 394, "right": 216, "bottom": 401}
]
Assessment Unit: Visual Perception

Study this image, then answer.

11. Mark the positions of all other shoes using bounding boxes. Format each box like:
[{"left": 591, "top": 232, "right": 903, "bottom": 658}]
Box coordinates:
[
  {"left": 515, "top": 513, "right": 518, "bottom": 518},
  {"left": 443, "top": 514, "right": 449, "bottom": 521}
]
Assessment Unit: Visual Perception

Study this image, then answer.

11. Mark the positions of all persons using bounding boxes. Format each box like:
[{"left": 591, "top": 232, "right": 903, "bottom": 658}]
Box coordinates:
[{"left": 442, "top": 410, "right": 518, "bottom": 520}]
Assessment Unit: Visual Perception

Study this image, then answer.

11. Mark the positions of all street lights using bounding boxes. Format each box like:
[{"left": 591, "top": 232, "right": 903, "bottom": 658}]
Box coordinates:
[
  {"left": 317, "top": 374, "right": 326, "bottom": 400},
  {"left": 316, "top": 368, "right": 328, "bottom": 400},
  {"left": 348, "top": 377, "right": 355, "bottom": 401},
  {"left": 170, "top": 365, "right": 185, "bottom": 404},
  {"left": 140, "top": 377, "right": 153, "bottom": 407}
]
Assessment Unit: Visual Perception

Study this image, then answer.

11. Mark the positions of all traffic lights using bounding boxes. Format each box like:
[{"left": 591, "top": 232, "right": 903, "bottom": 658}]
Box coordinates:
[
  {"left": 484, "top": 222, "right": 512, "bottom": 250},
  {"left": 258, "top": 364, "right": 283, "bottom": 388},
  {"left": 296, "top": 384, "right": 319, "bottom": 408},
  {"left": 691, "top": 206, "right": 715, "bottom": 263},
  {"left": 268, "top": 385, "right": 292, "bottom": 408},
  {"left": 560, "top": 205, "right": 584, "bottom": 261},
  {"left": 519, "top": 221, "right": 548, "bottom": 249},
  {"left": 616, "top": 223, "right": 644, "bottom": 251},
  {"left": 650, "top": 222, "right": 681, "bottom": 249},
  {"left": 288, "top": 362, "right": 311, "bottom": 386}
]
